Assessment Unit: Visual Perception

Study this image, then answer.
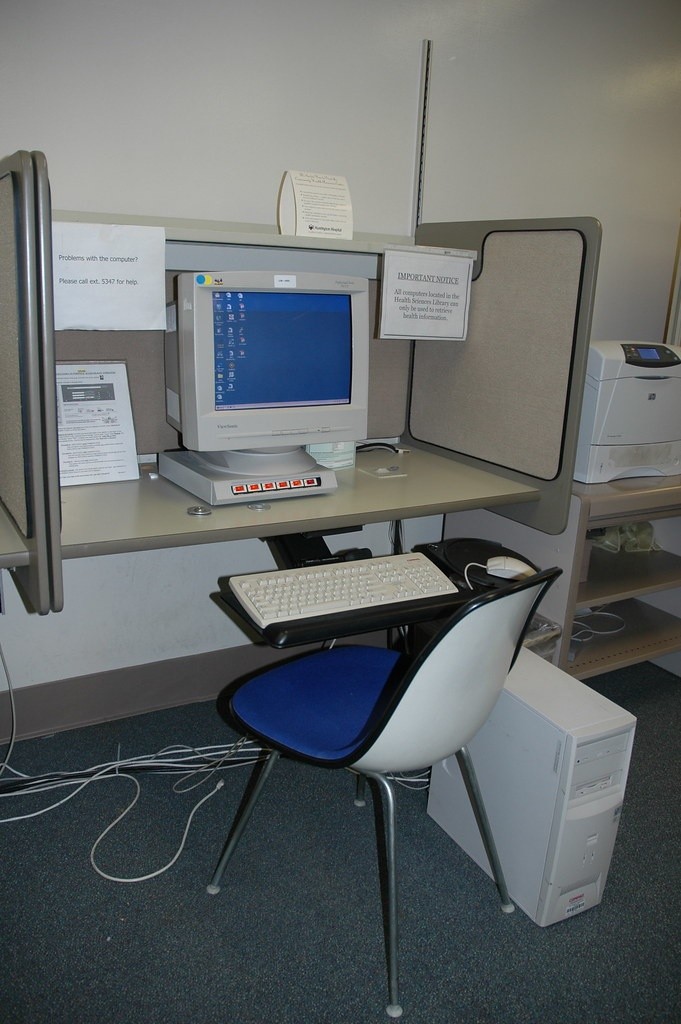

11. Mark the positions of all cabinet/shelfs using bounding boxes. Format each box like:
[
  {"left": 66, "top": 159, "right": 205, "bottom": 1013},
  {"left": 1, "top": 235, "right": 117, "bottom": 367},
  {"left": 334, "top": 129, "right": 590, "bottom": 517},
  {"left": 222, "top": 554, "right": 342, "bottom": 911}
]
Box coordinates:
[{"left": 440, "top": 478, "right": 681, "bottom": 681}]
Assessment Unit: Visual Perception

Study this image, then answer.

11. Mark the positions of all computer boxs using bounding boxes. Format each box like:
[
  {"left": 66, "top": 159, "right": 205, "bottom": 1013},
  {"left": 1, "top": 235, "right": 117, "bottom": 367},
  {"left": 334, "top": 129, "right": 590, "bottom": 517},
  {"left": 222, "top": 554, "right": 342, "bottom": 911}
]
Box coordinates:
[{"left": 426, "top": 646, "right": 637, "bottom": 928}]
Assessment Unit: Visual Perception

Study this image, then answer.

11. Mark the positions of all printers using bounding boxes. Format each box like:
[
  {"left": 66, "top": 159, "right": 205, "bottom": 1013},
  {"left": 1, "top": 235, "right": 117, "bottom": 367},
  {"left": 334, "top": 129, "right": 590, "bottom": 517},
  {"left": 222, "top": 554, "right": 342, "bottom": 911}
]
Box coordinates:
[{"left": 573, "top": 340, "right": 681, "bottom": 483}]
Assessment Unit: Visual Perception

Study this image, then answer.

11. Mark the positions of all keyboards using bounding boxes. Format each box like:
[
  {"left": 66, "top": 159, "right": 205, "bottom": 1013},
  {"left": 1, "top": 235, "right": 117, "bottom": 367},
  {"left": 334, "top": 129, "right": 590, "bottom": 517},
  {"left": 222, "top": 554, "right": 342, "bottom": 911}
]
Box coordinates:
[{"left": 228, "top": 552, "right": 459, "bottom": 628}]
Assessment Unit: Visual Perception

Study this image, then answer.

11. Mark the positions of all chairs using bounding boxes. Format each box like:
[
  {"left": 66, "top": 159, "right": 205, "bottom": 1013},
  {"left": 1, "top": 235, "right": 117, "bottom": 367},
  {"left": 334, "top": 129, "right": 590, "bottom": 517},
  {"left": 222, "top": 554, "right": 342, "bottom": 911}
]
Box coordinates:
[{"left": 206, "top": 565, "right": 563, "bottom": 1018}]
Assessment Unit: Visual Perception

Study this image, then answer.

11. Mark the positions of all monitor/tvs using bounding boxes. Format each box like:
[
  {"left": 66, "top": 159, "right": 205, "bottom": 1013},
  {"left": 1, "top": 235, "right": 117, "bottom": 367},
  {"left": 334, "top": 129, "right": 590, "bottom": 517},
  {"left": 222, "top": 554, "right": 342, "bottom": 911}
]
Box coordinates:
[{"left": 158, "top": 271, "right": 369, "bottom": 506}]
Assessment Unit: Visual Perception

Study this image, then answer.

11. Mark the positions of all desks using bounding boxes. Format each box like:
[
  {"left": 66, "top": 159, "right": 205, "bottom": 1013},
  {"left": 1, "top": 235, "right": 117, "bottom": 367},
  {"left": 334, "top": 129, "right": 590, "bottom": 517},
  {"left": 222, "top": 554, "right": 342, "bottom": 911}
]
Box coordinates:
[
  {"left": 0, "top": 149, "right": 50, "bottom": 616},
  {"left": 30, "top": 148, "right": 603, "bottom": 613}
]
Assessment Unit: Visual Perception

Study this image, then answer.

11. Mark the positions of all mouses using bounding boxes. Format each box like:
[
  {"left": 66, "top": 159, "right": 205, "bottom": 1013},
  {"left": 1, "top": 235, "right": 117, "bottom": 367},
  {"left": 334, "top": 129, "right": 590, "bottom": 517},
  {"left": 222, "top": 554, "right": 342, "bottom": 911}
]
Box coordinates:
[{"left": 486, "top": 556, "right": 537, "bottom": 580}]
